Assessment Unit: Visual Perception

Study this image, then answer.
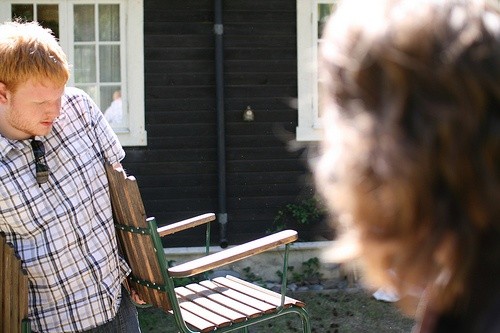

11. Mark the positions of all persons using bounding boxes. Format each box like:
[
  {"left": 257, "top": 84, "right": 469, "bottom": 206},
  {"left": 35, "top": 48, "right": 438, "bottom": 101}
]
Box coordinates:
[
  {"left": 308, "top": 0, "right": 500, "bottom": 333},
  {"left": 103, "top": 90, "right": 122, "bottom": 126},
  {"left": 0, "top": 20, "right": 146, "bottom": 333}
]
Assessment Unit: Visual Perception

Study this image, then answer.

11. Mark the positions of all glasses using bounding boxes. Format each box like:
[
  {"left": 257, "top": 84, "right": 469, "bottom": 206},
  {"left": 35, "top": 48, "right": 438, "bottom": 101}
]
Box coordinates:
[{"left": 30, "top": 136, "right": 49, "bottom": 188}]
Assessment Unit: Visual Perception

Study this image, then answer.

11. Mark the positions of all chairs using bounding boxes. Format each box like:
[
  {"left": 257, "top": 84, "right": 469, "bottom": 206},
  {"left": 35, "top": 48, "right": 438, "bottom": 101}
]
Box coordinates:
[
  {"left": 104, "top": 160, "right": 311, "bottom": 333},
  {"left": 0, "top": 230, "right": 31, "bottom": 333}
]
